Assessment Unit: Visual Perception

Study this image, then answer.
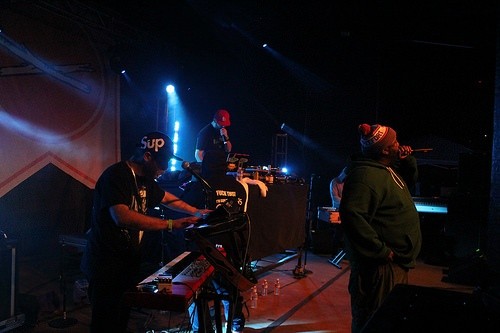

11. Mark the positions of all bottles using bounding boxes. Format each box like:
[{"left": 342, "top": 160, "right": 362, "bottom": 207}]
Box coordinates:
[
  {"left": 253, "top": 171, "right": 260, "bottom": 181},
  {"left": 266, "top": 170, "right": 274, "bottom": 184},
  {"left": 274, "top": 278, "right": 280, "bottom": 295},
  {"left": 237, "top": 167, "right": 243, "bottom": 180},
  {"left": 250, "top": 286, "right": 258, "bottom": 308},
  {"left": 261, "top": 279, "right": 268, "bottom": 296}
]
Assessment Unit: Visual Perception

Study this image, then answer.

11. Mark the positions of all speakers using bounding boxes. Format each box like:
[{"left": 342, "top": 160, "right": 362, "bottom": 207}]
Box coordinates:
[{"left": 359, "top": 284, "right": 500, "bottom": 333}]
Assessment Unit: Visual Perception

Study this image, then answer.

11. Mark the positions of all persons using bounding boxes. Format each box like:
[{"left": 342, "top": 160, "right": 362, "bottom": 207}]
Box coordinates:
[
  {"left": 174, "top": 145, "right": 269, "bottom": 333},
  {"left": 195, "top": 109, "right": 232, "bottom": 163},
  {"left": 334, "top": 124, "right": 422, "bottom": 333},
  {"left": 78, "top": 131, "right": 214, "bottom": 333},
  {"left": 329, "top": 166, "right": 346, "bottom": 210}
]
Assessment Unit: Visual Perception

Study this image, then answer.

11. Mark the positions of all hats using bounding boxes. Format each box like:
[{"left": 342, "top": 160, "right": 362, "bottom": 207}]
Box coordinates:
[
  {"left": 358, "top": 123, "right": 396, "bottom": 150},
  {"left": 137, "top": 126, "right": 174, "bottom": 157},
  {"left": 214, "top": 109, "right": 232, "bottom": 127}
]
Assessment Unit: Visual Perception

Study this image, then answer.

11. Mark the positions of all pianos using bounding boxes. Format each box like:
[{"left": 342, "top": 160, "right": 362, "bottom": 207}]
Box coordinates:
[
  {"left": 118, "top": 242, "right": 227, "bottom": 312},
  {"left": 182, "top": 193, "right": 249, "bottom": 239}
]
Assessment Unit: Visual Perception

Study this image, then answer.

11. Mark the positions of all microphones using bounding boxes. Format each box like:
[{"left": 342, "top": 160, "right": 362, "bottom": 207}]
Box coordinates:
[
  {"left": 401, "top": 148, "right": 433, "bottom": 156},
  {"left": 222, "top": 135, "right": 227, "bottom": 145},
  {"left": 181, "top": 161, "right": 211, "bottom": 189}
]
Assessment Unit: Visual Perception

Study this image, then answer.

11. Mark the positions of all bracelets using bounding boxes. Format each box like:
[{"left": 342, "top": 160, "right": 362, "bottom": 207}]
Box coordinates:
[
  {"left": 167, "top": 218, "right": 173, "bottom": 233},
  {"left": 226, "top": 137, "right": 230, "bottom": 141}
]
